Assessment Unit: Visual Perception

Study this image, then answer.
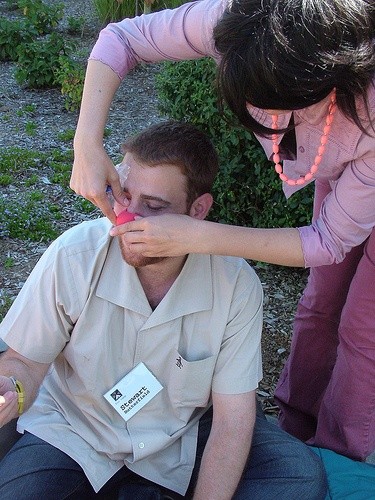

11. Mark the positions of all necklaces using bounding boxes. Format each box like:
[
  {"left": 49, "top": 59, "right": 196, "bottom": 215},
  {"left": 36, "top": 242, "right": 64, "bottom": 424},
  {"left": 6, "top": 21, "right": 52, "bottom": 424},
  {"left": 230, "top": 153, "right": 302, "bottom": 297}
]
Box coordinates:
[{"left": 272, "top": 87, "right": 337, "bottom": 186}]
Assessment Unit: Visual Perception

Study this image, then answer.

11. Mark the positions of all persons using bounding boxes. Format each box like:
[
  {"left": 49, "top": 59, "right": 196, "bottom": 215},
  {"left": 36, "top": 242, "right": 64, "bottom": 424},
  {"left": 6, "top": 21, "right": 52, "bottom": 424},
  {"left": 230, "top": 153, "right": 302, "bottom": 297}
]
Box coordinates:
[
  {"left": 0, "top": 121, "right": 328, "bottom": 500},
  {"left": 70, "top": 1, "right": 375, "bottom": 462}
]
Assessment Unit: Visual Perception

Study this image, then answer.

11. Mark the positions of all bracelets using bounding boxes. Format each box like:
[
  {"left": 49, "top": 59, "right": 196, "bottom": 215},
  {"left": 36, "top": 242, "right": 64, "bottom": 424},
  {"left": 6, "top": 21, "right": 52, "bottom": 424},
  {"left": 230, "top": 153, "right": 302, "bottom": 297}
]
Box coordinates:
[{"left": 8, "top": 376, "right": 25, "bottom": 418}]
